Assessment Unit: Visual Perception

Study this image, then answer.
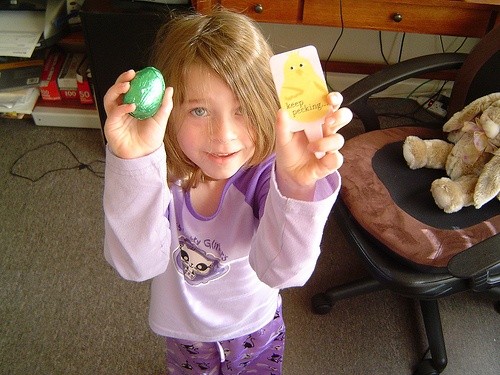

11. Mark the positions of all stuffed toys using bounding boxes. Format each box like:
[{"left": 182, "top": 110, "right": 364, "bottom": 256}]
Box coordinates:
[{"left": 403, "top": 92, "right": 500, "bottom": 214}]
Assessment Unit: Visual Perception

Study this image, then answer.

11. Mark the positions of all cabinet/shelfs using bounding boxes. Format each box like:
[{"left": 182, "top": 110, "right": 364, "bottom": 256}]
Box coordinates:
[{"left": 80, "top": 1, "right": 188, "bottom": 144}]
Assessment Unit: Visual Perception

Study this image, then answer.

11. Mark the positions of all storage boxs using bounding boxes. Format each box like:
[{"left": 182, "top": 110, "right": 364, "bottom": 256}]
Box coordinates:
[
  {"left": 39, "top": 54, "right": 64, "bottom": 101},
  {"left": 77, "top": 75, "right": 94, "bottom": 105},
  {"left": 57, "top": 53, "right": 84, "bottom": 99}
]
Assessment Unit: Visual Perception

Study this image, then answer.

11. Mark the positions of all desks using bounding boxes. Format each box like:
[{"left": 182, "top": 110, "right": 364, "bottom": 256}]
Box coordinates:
[{"left": 191, "top": 0, "right": 500, "bottom": 39}]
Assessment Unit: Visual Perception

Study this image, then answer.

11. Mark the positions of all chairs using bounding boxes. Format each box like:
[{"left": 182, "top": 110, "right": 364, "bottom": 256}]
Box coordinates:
[{"left": 311, "top": 24, "right": 500, "bottom": 375}]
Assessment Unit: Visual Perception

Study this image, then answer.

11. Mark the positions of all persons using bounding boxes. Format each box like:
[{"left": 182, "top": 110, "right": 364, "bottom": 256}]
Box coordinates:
[{"left": 103, "top": 6, "right": 353, "bottom": 375}]
